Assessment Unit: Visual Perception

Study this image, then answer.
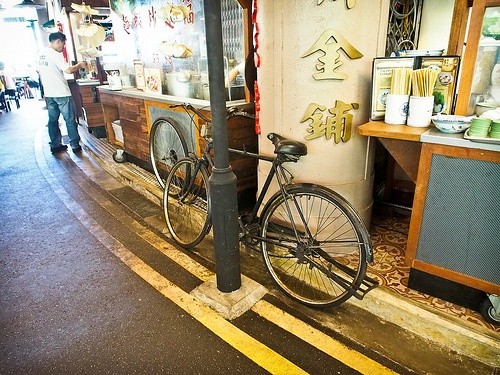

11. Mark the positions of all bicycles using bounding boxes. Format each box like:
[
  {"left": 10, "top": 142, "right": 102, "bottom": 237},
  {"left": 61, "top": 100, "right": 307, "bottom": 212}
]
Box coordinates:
[{"left": 163, "top": 103, "right": 379, "bottom": 309}]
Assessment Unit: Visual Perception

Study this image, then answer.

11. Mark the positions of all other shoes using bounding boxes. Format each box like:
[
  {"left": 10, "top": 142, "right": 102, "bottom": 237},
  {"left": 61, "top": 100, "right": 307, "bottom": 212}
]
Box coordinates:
[
  {"left": 50, "top": 144, "right": 68, "bottom": 152},
  {"left": 72, "top": 144, "right": 81, "bottom": 154}
]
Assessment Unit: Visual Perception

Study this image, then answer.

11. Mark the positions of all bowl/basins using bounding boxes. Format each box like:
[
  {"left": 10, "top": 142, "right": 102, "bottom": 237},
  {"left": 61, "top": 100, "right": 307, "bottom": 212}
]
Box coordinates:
[
  {"left": 432, "top": 114, "right": 472, "bottom": 134},
  {"left": 468, "top": 117, "right": 500, "bottom": 139},
  {"left": 475, "top": 103, "right": 495, "bottom": 116}
]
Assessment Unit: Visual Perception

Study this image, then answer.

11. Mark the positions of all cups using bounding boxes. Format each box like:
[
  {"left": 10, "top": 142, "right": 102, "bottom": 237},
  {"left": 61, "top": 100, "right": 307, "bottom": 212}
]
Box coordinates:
[
  {"left": 407, "top": 96, "right": 434, "bottom": 128},
  {"left": 384, "top": 94, "right": 410, "bottom": 125}
]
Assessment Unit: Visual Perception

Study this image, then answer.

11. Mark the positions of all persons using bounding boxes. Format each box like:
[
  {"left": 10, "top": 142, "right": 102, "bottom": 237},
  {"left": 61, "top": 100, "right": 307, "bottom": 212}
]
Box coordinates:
[
  {"left": 35, "top": 31, "right": 86, "bottom": 152},
  {"left": 0, "top": 61, "right": 15, "bottom": 110},
  {"left": 225, "top": 60, "right": 245, "bottom": 88}
]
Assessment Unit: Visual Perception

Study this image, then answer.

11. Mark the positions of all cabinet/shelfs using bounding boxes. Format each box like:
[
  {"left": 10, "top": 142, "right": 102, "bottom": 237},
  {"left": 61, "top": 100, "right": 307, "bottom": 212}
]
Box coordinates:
[
  {"left": 98, "top": 85, "right": 257, "bottom": 195},
  {"left": 75, "top": 79, "right": 104, "bottom": 134},
  {"left": 357, "top": 121, "right": 500, "bottom": 298}
]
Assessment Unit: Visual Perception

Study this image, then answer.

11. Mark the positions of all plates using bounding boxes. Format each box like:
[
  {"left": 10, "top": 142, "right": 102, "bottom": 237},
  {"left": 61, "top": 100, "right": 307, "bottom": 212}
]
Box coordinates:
[{"left": 404, "top": 49, "right": 445, "bottom": 57}]
